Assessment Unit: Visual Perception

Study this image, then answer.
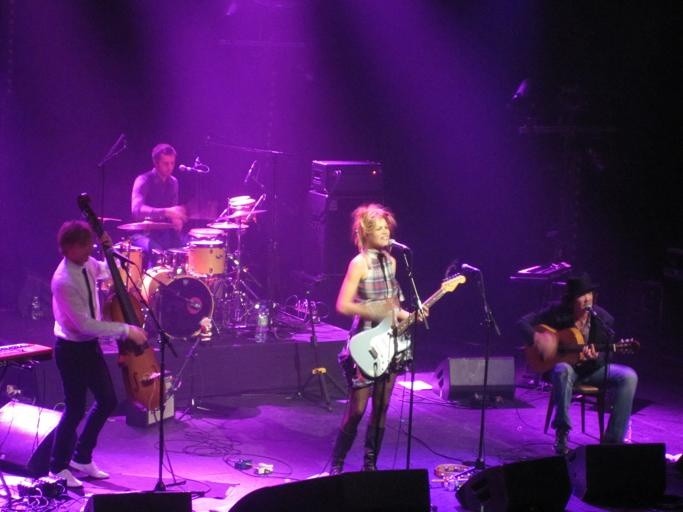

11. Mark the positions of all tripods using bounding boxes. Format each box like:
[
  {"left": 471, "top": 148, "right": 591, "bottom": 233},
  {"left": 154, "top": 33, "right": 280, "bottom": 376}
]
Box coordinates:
[
  {"left": 292, "top": 308, "right": 349, "bottom": 414},
  {"left": 448, "top": 366, "right": 500, "bottom": 476},
  {"left": 222, "top": 248, "right": 270, "bottom": 329}
]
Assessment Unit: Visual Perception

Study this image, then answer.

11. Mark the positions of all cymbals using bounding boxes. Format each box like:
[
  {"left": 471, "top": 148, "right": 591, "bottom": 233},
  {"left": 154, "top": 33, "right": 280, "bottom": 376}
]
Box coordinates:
[
  {"left": 213, "top": 209, "right": 267, "bottom": 220},
  {"left": 115, "top": 221, "right": 175, "bottom": 231},
  {"left": 206, "top": 221, "right": 249, "bottom": 230},
  {"left": 96, "top": 217, "right": 122, "bottom": 222}
]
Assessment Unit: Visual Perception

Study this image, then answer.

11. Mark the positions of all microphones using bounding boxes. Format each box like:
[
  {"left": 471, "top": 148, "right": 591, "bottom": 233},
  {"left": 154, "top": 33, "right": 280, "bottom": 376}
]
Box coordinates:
[
  {"left": 577, "top": 303, "right": 614, "bottom": 338},
  {"left": 189, "top": 298, "right": 201, "bottom": 311},
  {"left": 388, "top": 238, "right": 413, "bottom": 254},
  {"left": 178, "top": 164, "right": 203, "bottom": 174},
  {"left": 108, "top": 246, "right": 141, "bottom": 273}
]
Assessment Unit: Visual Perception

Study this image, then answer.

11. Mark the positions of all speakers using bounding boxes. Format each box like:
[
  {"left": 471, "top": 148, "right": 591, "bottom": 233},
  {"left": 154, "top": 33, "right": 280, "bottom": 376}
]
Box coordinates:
[
  {"left": 433, "top": 355, "right": 516, "bottom": 401},
  {"left": 454, "top": 455, "right": 573, "bottom": 512},
  {"left": 566, "top": 442, "right": 667, "bottom": 507},
  {"left": 83, "top": 492, "right": 192, "bottom": 512},
  {"left": 227, "top": 468, "right": 431, "bottom": 512},
  {"left": 0, "top": 400, "right": 78, "bottom": 477}
]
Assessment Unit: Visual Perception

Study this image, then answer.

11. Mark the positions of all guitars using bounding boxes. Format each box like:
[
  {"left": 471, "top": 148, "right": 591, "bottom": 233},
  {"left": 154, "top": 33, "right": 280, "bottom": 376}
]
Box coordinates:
[
  {"left": 523, "top": 323, "right": 640, "bottom": 374},
  {"left": 349, "top": 272, "right": 466, "bottom": 379}
]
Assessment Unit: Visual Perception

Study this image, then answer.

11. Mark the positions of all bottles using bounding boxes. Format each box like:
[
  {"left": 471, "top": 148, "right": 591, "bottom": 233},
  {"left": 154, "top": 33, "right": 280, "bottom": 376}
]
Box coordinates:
[
  {"left": 31, "top": 294, "right": 41, "bottom": 320},
  {"left": 440, "top": 464, "right": 471, "bottom": 491},
  {"left": 256, "top": 304, "right": 270, "bottom": 340},
  {"left": 625, "top": 418, "right": 633, "bottom": 444}
]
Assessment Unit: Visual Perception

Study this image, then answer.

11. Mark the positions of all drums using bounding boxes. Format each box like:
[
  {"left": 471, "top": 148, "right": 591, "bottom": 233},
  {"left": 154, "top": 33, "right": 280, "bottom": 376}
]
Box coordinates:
[
  {"left": 183, "top": 239, "right": 225, "bottom": 278},
  {"left": 161, "top": 248, "right": 188, "bottom": 273},
  {"left": 188, "top": 228, "right": 228, "bottom": 248},
  {"left": 100, "top": 243, "right": 144, "bottom": 293},
  {"left": 140, "top": 265, "right": 214, "bottom": 340}
]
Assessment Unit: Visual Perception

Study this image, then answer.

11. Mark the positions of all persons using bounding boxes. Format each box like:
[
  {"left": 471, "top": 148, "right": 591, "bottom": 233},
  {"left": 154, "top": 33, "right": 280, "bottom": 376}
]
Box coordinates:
[
  {"left": 46, "top": 221, "right": 150, "bottom": 488},
  {"left": 129, "top": 141, "right": 188, "bottom": 264},
  {"left": 515, "top": 270, "right": 639, "bottom": 454},
  {"left": 328, "top": 200, "right": 429, "bottom": 476}
]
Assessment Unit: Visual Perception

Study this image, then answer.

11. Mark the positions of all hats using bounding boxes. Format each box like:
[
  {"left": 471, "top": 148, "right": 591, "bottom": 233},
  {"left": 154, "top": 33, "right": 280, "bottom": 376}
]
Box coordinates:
[{"left": 563, "top": 270, "right": 601, "bottom": 298}]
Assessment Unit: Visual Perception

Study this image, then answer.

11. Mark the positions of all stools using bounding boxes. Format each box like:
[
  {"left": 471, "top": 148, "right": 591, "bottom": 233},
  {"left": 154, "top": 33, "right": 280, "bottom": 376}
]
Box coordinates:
[{"left": 538, "top": 380, "right": 605, "bottom": 444}]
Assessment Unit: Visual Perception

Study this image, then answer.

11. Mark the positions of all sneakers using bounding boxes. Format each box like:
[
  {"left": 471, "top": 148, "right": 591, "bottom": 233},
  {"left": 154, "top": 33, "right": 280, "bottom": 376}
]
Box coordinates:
[
  {"left": 551, "top": 429, "right": 570, "bottom": 457},
  {"left": 46, "top": 467, "right": 84, "bottom": 489},
  {"left": 69, "top": 457, "right": 110, "bottom": 479}
]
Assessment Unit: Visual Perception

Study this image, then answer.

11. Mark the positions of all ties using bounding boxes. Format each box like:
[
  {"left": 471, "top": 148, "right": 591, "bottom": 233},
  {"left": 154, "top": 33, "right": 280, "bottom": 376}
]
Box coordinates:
[{"left": 81, "top": 267, "right": 97, "bottom": 321}]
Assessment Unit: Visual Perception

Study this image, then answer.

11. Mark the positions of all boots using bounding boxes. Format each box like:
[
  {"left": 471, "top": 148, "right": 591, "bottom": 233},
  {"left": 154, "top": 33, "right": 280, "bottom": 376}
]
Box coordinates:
[
  {"left": 327, "top": 426, "right": 358, "bottom": 476},
  {"left": 360, "top": 424, "right": 386, "bottom": 471}
]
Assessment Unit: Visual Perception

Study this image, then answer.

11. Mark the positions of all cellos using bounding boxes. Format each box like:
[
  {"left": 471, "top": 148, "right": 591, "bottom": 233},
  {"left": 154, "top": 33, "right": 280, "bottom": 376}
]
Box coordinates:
[{"left": 76, "top": 192, "right": 167, "bottom": 412}]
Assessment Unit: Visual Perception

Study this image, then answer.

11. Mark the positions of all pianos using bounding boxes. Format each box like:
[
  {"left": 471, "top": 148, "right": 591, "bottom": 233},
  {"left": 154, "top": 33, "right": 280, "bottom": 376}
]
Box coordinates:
[{"left": 0, "top": 342, "right": 53, "bottom": 368}]
